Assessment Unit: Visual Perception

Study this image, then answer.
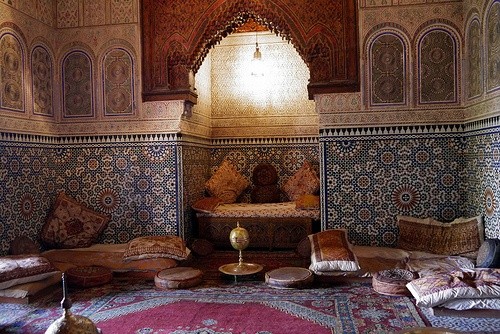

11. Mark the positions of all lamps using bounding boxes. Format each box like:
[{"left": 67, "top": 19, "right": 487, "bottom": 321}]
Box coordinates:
[{"left": 251, "top": 33, "right": 265, "bottom": 76}]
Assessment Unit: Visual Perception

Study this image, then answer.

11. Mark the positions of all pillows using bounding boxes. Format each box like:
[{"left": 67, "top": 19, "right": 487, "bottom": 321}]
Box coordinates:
[
  {"left": 41, "top": 193, "right": 111, "bottom": 249},
  {"left": 205, "top": 159, "right": 251, "bottom": 203},
  {"left": 398, "top": 216, "right": 483, "bottom": 255},
  {"left": 308, "top": 229, "right": 360, "bottom": 273},
  {"left": 0, "top": 254, "right": 61, "bottom": 293},
  {"left": 121, "top": 235, "right": 189, "bottom": 266},
  {"left": 281, "top": 161, "right": 320, "bottom": 201}
]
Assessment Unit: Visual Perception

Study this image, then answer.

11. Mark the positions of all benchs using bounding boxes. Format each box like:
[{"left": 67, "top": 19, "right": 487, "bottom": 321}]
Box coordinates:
[
  {"left": 41, "top": 244, "right": 174, "bottom": 279},
  {"left": 193, "top": 198, "right": 320, "bottom": 250}
]
